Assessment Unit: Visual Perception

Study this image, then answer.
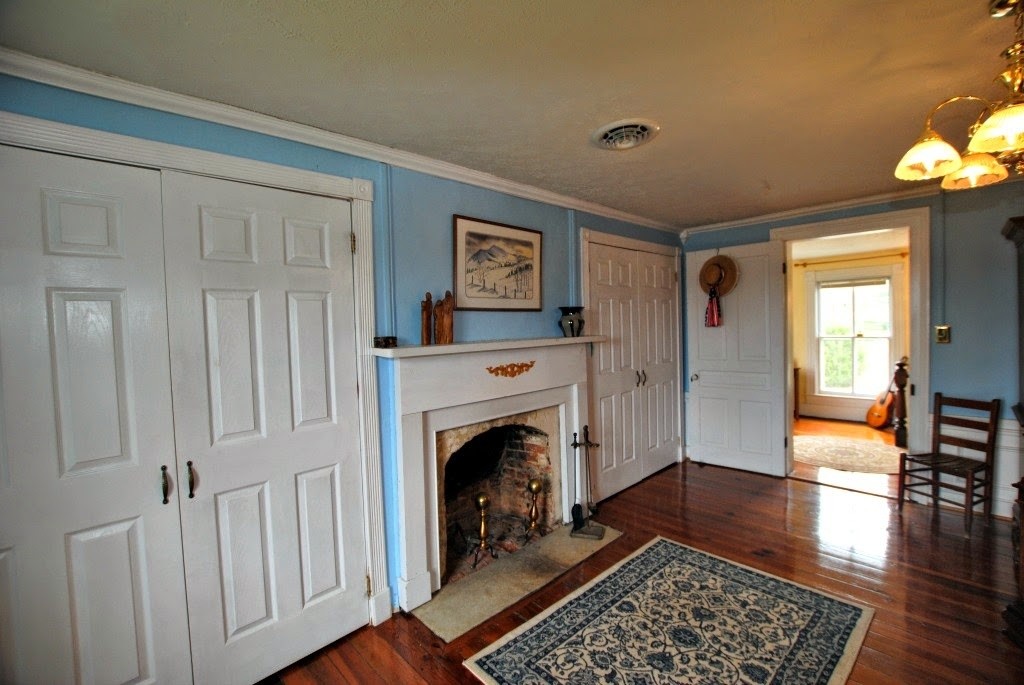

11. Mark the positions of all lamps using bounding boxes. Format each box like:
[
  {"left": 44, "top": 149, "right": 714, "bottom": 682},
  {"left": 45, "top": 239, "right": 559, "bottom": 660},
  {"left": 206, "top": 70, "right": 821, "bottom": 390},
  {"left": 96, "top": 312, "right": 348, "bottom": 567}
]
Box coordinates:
[{"left": 894, "top": 0, "right": 1024, "bottom": 190}]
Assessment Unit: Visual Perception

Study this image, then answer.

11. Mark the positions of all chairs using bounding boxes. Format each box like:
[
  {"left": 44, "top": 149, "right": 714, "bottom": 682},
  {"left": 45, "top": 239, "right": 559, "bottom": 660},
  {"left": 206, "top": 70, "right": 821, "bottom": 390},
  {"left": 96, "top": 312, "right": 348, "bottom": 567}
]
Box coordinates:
[{"left": 897, "top": 393, "right": 1000, "bottom": 540}]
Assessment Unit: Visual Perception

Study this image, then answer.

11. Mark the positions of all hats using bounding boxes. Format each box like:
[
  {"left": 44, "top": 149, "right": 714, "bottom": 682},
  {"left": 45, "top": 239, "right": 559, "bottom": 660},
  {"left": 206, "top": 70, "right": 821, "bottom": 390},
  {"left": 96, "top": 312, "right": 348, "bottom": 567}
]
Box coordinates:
[{"left": 698, "top": 254, "right": 739, "bottom": 328}]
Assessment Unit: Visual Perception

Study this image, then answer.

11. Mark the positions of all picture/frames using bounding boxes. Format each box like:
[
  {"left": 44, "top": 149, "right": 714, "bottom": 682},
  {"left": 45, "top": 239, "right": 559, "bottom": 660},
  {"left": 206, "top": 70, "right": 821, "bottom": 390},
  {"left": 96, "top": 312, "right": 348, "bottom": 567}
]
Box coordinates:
[{"left": 452, "top": 212, "right": 544, "bottom": 312}]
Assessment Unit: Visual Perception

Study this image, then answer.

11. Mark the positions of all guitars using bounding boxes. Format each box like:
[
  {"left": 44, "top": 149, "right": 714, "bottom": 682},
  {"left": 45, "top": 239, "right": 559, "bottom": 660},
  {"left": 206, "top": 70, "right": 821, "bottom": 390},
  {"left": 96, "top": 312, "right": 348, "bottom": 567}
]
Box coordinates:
[{"left": 866, "top": 356, "right": 909, "bottom": 428}]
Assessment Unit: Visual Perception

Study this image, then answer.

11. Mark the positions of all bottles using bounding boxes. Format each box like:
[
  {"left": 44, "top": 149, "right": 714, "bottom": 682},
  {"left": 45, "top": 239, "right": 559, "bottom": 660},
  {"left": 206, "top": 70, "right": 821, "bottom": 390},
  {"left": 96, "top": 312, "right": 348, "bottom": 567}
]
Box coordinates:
[{"left": 557, "top": 306, "right": 585, "bottom": 337}]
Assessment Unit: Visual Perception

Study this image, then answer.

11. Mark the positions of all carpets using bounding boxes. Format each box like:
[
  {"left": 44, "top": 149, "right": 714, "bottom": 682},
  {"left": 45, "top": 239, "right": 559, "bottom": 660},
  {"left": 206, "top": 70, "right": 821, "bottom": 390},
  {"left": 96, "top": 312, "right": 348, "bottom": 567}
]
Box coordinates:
[
  {"left": 463, "top": 536, "right": 874, "bottom": 685},
  {"left": 793, "top": 434, "right": 907, "bottom": 474}
]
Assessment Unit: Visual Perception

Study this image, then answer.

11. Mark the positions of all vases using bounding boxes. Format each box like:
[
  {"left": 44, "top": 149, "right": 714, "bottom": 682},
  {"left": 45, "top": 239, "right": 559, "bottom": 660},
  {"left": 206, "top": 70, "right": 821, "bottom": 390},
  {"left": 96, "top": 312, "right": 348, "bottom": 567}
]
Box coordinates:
[{"left": 558, "top": 306, "right": 585, "bottom": 337}]
